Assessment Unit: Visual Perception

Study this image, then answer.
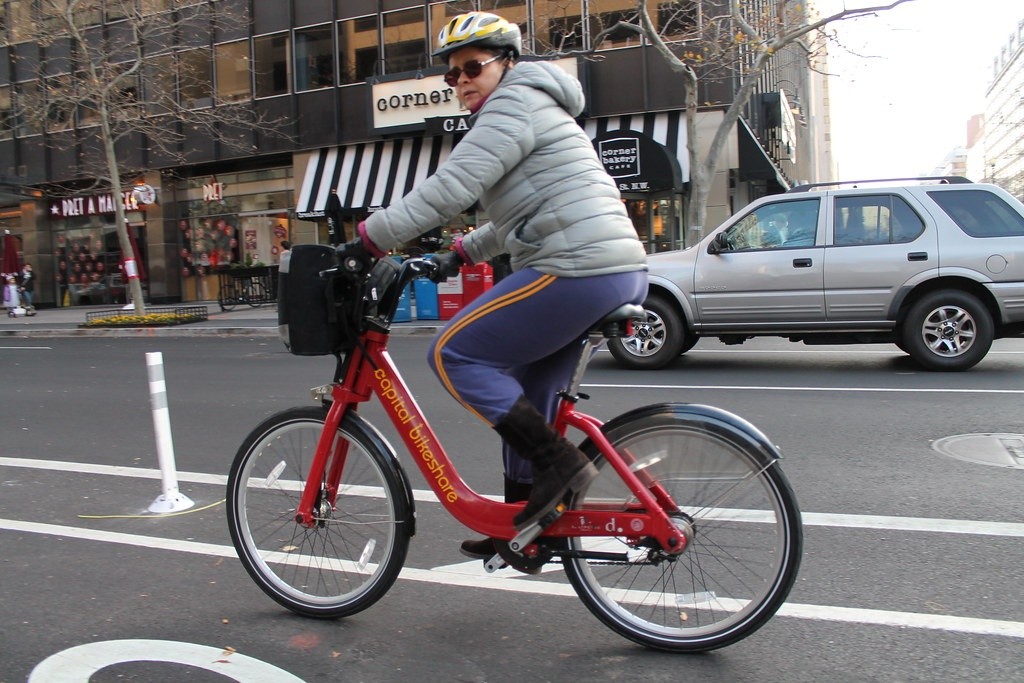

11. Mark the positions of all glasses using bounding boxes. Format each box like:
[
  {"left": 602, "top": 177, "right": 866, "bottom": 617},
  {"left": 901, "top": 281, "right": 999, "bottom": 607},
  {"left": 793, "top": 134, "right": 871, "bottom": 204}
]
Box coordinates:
[{"left": 444, "top": 51, "right": 508, "bottom": 87}]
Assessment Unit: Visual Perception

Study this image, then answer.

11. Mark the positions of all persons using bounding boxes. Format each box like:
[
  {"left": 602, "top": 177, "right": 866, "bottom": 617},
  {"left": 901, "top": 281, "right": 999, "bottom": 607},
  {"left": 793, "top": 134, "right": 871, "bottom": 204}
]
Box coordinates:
[
  {"left": 0, "top": 262, "right": 37, "bottom": 317},
  {"left": 759, "top": 204, "right": 817, "bottom": 248},
  {"left": 357, "top": 11, "right": 652, "bottom": 577}
]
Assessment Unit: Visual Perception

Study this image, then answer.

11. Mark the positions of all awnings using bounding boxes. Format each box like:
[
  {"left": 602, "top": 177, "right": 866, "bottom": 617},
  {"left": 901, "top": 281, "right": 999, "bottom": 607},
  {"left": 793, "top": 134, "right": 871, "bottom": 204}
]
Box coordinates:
[{"left": 293, "top": 110, "right": 692, "bottom": 225}]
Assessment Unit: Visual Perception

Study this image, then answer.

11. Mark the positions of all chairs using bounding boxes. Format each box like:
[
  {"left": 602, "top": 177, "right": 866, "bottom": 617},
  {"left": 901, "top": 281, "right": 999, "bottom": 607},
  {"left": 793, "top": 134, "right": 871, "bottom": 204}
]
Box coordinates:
[{"left": 838, "top": 215, "right": 864, "bottom": 244}]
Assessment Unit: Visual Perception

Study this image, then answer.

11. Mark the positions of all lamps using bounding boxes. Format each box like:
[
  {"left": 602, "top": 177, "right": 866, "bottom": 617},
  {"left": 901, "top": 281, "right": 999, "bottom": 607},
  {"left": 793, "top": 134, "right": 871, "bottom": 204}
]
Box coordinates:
[{"left": 771, "top": 79, "right": 807, "bottom": 128}]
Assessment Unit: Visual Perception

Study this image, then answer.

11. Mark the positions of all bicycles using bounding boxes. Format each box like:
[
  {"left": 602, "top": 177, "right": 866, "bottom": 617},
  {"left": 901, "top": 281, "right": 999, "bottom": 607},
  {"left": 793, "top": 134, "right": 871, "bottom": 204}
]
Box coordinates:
[
  {"left": 216, "top": 269, "right": 265, "bottom": 311},
  {"left": 226, "top": 244, "right": 804, "bottom": 653}
]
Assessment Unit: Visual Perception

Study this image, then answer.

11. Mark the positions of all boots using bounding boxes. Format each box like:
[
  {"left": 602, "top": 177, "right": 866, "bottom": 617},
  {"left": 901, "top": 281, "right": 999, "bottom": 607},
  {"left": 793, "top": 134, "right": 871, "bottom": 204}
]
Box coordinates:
[
  {"left": 459, "top": 472, "right": 542, "bottom": 575},
  {"left": 491, "top": 394, "right": 599, "bottom": 531}
]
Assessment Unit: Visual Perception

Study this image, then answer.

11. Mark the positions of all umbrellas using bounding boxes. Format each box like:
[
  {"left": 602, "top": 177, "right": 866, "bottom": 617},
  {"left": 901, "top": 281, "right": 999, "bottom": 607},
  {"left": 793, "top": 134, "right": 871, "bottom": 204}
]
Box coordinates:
[{"left": 323, "top": 188, "right": 348, "bottom": 248}]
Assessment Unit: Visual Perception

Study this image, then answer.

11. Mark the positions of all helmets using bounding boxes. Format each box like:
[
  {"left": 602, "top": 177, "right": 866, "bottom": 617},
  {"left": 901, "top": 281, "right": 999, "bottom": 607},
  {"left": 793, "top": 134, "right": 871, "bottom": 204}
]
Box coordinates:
[{"left": 431, "top": 12, "right": 521, "bottom": 65}]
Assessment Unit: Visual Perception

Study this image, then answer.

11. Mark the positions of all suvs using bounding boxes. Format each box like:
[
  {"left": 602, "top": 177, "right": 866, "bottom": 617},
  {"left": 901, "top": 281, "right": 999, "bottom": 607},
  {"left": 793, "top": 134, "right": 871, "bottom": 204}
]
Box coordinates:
[{"left": 602, "top": 174, "right": 1024, "bottom": 371}]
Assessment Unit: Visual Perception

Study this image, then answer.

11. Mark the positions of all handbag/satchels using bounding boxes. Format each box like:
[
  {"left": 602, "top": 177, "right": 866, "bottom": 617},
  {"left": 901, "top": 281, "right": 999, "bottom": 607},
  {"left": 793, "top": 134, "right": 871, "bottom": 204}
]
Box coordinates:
[{"left": 278, "top": 244, "right": 401, "bottom": 383}]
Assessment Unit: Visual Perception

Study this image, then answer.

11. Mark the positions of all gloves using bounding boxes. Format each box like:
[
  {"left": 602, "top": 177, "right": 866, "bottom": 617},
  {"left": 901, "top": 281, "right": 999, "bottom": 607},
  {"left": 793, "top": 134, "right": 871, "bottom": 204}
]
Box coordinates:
[
  {"left": 426, "top": 250, "right": 464, "bottom": 284},
  {"left": 337, "top": 239, "right": 373, "bottom": 281}
]
